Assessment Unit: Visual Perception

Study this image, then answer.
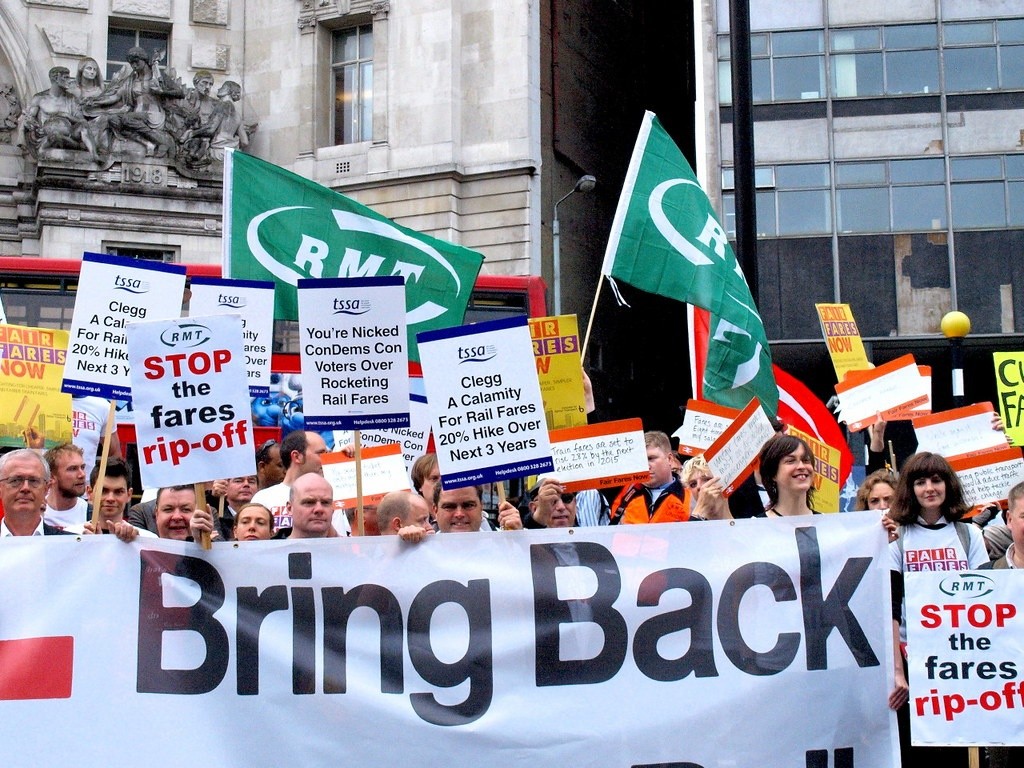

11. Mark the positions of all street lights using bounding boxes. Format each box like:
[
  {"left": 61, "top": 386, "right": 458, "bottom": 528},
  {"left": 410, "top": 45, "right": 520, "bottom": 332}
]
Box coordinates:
[{"left": 553, "top": 174, "right": 597, "bottom": 317}]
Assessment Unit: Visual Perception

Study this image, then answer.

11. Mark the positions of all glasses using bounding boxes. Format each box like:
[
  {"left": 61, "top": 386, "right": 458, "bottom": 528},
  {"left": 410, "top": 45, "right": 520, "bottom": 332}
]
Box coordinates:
[
  {"left": 552, "top": 493, "right": 575, "bottom": 506},
  {"left": 0, "top": 475, "right": 47, "bottom": 488},
  {"left": 256, "top": 439, "right": 275, "bottom": 458}
]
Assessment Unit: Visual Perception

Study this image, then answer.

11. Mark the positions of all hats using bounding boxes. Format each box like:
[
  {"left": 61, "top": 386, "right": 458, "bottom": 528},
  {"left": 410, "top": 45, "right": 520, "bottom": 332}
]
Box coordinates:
[{"left": 528, "top": 477, "right": 546, "bottom": 501}]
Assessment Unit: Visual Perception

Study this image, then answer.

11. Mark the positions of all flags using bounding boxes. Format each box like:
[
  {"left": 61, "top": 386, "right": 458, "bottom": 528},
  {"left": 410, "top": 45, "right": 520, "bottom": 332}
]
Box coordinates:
[
  {"left": 686, "top": 302, "right": 855, "bottom": 488},
  {"left": 602, "top": 111, "right": 780, "bottom": 419},
  {"left": 222, "top": 148, "right": 485, "bottom": 366}
]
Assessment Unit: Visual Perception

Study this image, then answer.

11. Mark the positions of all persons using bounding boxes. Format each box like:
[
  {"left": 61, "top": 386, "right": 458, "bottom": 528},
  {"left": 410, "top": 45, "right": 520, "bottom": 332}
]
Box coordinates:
[
  {"left": 375, "top": 417, "right": 815, "bottom": 545},
  {"left": 854, "top": 410, "right": 1024, "bottom": 768},
  {"left": 0, "top": 45, "right": 249, "bottom": 171},
  {"left": 0, "top": 396, "right": 357, "bottom": 541}
]
